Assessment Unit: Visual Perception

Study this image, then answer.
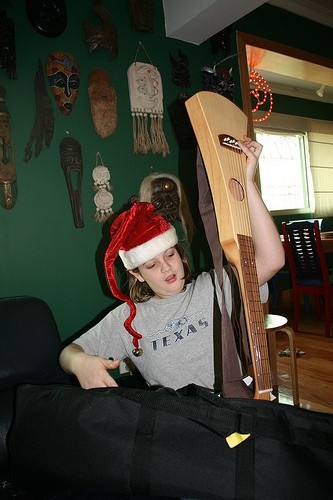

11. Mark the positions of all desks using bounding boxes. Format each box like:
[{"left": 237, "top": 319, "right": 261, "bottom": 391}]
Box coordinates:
[{"left": 280, "top": 231, "right": 333, "bottom": 253}]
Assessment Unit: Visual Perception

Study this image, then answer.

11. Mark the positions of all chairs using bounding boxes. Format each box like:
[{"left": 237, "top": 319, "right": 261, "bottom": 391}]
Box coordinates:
[
  {"left": 0, "top": 296, "right": 73, "bottom": 474},
  {"left": 282, "top": 220, "right": 333, "bottom": 337}
]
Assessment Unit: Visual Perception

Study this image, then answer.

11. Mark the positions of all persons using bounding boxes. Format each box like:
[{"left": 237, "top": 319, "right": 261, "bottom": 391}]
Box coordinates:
[{"left": 59, "top": 135, "right": 285, "bottom": 389}]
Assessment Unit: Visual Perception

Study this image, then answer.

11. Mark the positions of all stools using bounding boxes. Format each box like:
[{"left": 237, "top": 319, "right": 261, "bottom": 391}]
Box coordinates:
[{"left": 264, "top": 314, "right": 300, "bottom": 405}]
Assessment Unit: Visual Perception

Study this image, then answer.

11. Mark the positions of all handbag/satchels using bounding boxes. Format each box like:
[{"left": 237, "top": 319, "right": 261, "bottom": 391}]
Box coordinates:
[{"left": 0, "top": 385, "right": 333, "bottom": 500}]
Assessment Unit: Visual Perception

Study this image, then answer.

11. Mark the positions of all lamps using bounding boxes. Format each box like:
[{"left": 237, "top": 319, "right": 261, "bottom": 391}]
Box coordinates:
[{"left": 316, "top": 85, "right": 327, "bottom": 97}]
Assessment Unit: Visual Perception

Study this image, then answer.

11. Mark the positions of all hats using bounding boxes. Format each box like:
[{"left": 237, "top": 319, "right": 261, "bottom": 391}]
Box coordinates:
[{"left": 103, "top": 201, "right": 179, "bottom": 357}]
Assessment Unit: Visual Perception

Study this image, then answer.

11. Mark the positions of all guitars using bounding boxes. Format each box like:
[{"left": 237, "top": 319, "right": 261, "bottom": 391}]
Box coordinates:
[{"left": 184, "top": 90, "right": 272, "bottom": 402}]
